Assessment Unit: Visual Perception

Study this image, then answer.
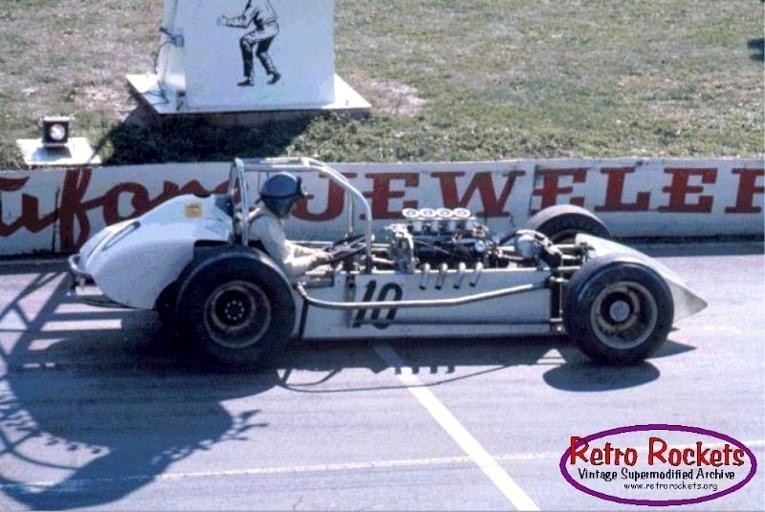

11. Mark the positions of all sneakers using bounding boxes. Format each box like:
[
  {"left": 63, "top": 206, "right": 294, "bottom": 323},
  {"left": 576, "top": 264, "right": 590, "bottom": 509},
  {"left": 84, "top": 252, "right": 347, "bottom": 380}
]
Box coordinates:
[
  {"left": 268, "top": 74, "right": 280, "bottom": 86},
  {"left": 238, "top": 79, "right": 256, "bottom": 88}
]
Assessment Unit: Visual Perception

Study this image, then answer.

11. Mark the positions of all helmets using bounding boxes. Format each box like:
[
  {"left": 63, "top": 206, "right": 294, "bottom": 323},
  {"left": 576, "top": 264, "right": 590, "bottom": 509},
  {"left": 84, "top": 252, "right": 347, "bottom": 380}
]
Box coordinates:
[{"left": 260, "top": 171, "right": 314, "bottom": 217}]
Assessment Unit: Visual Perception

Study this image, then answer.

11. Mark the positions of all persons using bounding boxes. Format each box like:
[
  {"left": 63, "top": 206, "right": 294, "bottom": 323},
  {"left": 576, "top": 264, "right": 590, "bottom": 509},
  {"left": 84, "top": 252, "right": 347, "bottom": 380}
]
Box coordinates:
[
  {"left": 215, "top": 1, "right": 282, "bottom": 87},
  {"left": 233, "top": 171, "right": 364, "bottom": 288}
]
